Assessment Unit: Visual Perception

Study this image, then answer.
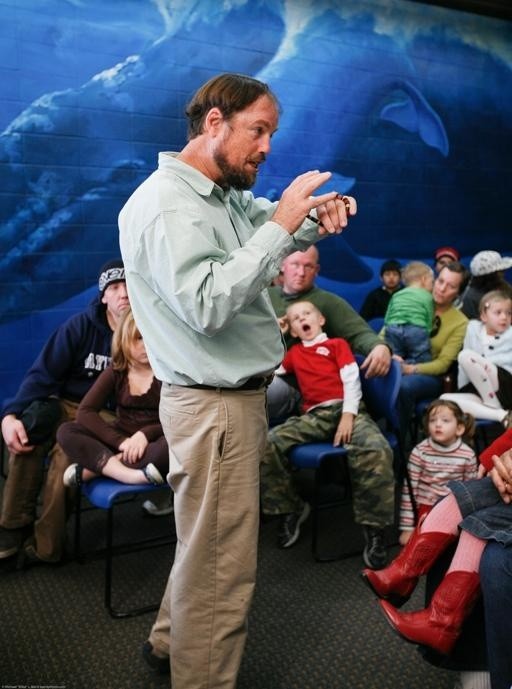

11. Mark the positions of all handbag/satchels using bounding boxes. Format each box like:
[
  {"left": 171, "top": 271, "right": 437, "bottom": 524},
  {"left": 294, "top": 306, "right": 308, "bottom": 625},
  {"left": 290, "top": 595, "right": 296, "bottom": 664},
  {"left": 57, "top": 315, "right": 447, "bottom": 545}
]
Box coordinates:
[{"left": 19, "top": 397, "right": 60, "bottom": 452}]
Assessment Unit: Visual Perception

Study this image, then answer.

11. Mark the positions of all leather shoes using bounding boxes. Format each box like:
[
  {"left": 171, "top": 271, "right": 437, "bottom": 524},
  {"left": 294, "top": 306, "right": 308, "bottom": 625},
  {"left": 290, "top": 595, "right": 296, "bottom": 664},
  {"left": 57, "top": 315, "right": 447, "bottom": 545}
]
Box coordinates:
[
  {"left": 143, "top": 464, "right": 164, "bottom": 487},
  {"left": 62, "top": 463, "right": 82, "bottom": 488}
]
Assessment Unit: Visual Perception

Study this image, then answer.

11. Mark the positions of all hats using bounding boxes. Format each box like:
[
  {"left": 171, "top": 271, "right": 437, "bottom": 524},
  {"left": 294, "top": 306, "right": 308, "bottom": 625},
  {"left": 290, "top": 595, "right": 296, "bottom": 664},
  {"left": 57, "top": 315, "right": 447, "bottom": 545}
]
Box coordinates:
[
  {"left": 436, "top": 248, "right": 458, "bottom": 262},
  {"left": 470, "top": 250, "right": 512, "bottom": 277},
  {"left": 99, "top": 264, "right": 127, "bottom": 301}
]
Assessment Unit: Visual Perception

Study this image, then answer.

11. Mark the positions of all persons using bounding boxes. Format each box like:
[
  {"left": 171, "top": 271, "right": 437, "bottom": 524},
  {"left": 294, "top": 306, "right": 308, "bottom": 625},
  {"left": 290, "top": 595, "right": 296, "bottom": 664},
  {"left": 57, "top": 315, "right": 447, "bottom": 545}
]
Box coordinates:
[
  {"left": 115, "top": 72, "right": 363, "bottom": 689},
  {"left": 364, "top": 426, "right": 512, "bottom": 656},
  {"left": 260, "top": 298, "right": 396, "bottom": 570},
  {"left": 57, "top": 306, "right": 169, "bottom": 488},
  {"left": 396, "top": 401, "right": 481, "bottom": 547},
  {"left": 423, "top": 518, "right": 511, "bottom": 687},
  {"left": 0, "top": 258, "right": 130, "bottom": 575},
  {"left": 266, "top": 243, "right": 512, "bottom": 427}
]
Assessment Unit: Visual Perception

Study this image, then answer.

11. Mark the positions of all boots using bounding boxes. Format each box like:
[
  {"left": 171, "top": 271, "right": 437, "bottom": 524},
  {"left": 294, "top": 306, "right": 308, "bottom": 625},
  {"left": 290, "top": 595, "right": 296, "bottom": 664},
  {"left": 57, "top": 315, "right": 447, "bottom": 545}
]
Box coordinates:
[
  {"left": 362, "top": 508, "right": 460, "bottom": 608},
  {"left": 378, "top": 569, "right": 481, "bottom": 653}
]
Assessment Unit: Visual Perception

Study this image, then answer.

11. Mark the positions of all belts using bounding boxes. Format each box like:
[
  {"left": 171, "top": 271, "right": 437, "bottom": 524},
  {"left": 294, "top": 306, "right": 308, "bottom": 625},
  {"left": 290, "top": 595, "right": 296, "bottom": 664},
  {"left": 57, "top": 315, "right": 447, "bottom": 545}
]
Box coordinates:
[{"left": 172, "top": 374, "right": 277, "bottom": 392}]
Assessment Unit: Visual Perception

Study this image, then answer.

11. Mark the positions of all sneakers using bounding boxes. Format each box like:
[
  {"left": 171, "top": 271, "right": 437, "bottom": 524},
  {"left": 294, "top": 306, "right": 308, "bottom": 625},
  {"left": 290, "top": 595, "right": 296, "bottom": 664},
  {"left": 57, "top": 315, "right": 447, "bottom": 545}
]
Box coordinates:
[
  {"left": 363, "top": 525, "right": 394, "bottom": 569},
  {"left": 0, "top": 529, "right": 20, "bottom": 560},
  {"left": 274, "top": 498, "right": 310, "bottom": 548},
  {"left": 142, "top": 638, "right": 170, "bottom": 668}
]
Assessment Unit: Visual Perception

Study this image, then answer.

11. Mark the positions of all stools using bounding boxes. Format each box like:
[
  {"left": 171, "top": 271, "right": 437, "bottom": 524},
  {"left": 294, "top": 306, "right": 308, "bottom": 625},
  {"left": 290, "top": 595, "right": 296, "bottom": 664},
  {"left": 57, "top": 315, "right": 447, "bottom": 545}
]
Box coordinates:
[
  {"left": 411, "top": 396, "right": 433, "bottom": 451},
  {"left": 70, "top": 477, "right": 175, "bottom": 622},
  {"left": 464, "top": 416, "right": 500, "bottom": 457}
]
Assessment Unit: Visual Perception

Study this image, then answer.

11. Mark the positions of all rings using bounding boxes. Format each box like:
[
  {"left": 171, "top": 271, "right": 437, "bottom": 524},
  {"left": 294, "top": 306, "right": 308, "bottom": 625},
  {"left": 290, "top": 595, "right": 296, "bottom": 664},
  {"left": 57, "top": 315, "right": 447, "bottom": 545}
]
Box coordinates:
[{"left": 504, "top": 481, "right": 509, "bottom": 490}]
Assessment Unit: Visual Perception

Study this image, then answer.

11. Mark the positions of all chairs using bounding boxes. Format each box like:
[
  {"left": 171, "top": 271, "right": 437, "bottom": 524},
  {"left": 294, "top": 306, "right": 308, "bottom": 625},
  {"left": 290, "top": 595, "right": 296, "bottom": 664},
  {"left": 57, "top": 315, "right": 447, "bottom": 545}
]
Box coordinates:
[
  {"left": 367, "top": 316, "right": 385, "bottom": 334},
  {"left": 291, "top": 358, "right": 409, "bottom": 568}
]
